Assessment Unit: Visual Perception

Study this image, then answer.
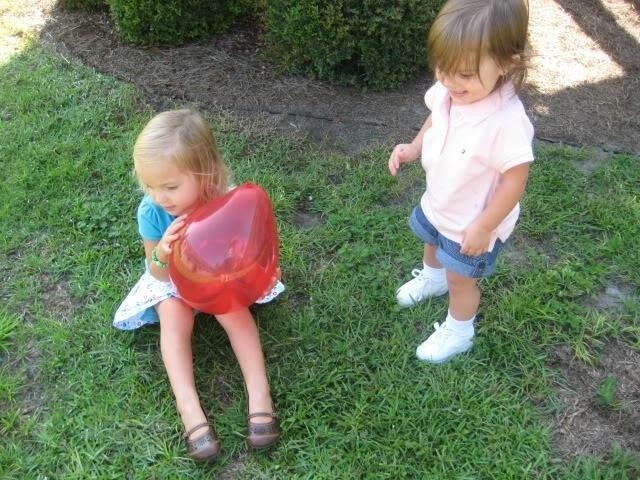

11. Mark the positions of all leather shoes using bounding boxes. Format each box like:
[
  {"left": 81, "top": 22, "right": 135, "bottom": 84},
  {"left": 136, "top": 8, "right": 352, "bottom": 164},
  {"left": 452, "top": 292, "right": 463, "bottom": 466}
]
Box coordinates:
[
  {"left": 176, "top": 405, "right": 221, "bottom": 462},
  {"left": 246, "top": 395, "right": 281, "bottom": 449}
]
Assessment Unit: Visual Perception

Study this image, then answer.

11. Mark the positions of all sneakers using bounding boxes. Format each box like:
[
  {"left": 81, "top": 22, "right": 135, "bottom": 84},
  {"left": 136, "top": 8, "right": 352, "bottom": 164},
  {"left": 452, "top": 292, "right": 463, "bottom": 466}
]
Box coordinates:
[
  {"left": 396, "top": 272, "right": 449, "bottom": 308},
  {"left": 418, "top": 322, "right": 475, "bottom": 362}
]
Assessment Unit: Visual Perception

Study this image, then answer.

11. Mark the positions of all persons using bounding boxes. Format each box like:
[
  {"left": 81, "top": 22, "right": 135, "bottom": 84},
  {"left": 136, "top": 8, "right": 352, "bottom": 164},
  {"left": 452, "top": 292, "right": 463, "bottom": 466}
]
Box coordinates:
[
  {"left": 113, "top": 107, "right": 286, "bottom": 464},
  {"left": 382, "top": 2, "right": 535, "bottom": 364}
]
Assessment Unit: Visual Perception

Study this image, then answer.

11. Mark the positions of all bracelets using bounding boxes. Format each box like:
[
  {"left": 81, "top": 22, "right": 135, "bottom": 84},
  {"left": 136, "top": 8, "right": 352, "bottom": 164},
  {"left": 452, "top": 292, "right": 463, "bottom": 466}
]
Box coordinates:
[{"left": 151, "top": 247, "right": 168, "bottom": 269}]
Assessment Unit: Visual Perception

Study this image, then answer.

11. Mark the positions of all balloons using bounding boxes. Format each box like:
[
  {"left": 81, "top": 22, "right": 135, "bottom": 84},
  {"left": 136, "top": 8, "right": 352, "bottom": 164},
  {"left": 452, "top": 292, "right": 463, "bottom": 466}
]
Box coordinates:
[{"left": 166, "top": 183, "right": 280, "bottom": 314}]
